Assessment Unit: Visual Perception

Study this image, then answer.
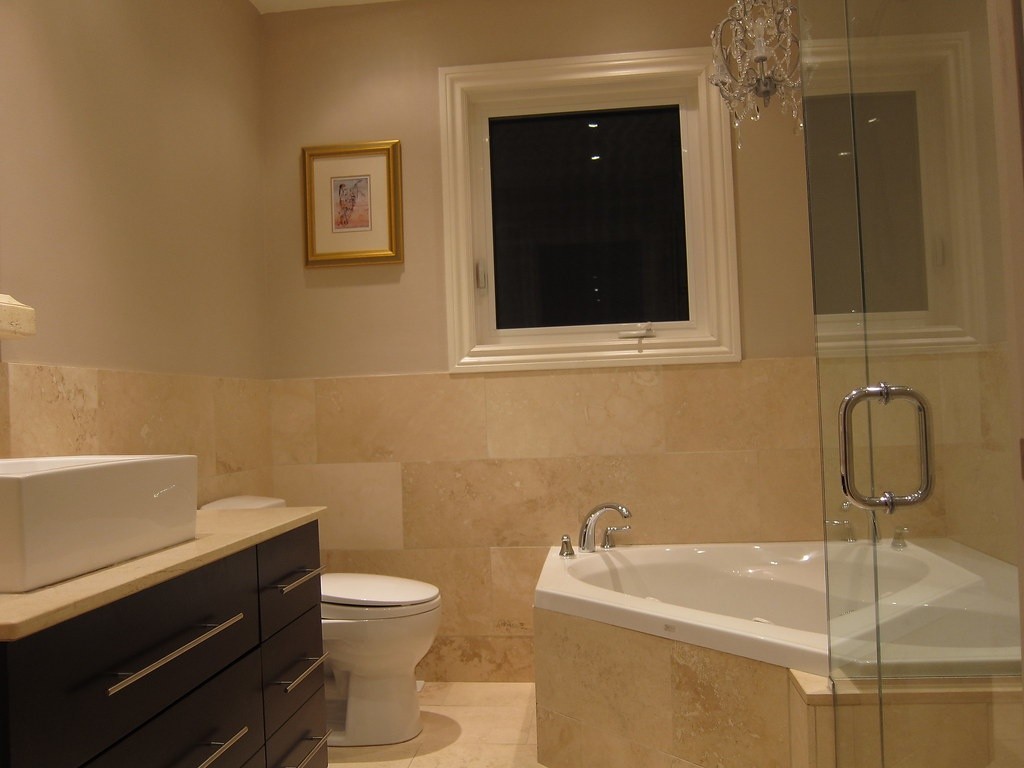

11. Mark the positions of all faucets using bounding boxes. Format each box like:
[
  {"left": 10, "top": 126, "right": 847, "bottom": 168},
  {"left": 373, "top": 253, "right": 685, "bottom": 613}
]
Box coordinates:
[
  {"left": 840, "top": 494, "right": 883, "bottom": 544},
  {"left": 580, "top": 502, "right": 631, "bottom": 553}
]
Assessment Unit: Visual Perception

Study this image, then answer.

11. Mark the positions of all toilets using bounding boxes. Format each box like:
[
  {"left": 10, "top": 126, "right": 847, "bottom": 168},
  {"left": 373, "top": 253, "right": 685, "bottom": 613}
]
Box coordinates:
[{"left": 200, "top": 491, "right": 445, "bottom": 745}]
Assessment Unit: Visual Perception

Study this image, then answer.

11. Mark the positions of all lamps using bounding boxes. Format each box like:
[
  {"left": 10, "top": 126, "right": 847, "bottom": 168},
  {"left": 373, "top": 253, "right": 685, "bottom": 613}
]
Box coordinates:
[{"left": 706, "top": 0, "right": 807, "bottom": 148}]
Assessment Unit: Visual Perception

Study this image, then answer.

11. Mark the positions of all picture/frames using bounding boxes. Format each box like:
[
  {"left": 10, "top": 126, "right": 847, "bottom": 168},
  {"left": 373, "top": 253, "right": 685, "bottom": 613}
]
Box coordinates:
[{"left": 300, "top": 140, "right": 405, "bottom": 270}]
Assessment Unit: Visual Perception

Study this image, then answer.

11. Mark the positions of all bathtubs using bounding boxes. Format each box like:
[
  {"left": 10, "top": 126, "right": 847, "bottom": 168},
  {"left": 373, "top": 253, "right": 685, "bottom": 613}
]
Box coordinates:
[
  {"left": 532, "top": 537, "right": 829, "bottom": 768},
  {"left": 829, "top": 530, "right": 1024, "bottom": 767}
]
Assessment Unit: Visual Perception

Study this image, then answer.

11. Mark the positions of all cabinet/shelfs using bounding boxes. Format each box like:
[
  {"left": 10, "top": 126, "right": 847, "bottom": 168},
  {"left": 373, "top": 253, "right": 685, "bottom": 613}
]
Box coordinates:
[{"left": 0, "top": 517, "right": 330, "bottom": 768}]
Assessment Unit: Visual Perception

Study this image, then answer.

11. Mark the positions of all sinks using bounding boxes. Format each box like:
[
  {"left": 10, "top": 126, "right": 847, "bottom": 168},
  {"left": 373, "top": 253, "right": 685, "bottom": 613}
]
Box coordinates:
[{"left": 0, "top": 451, "right": 198, "bottom": 596}]
